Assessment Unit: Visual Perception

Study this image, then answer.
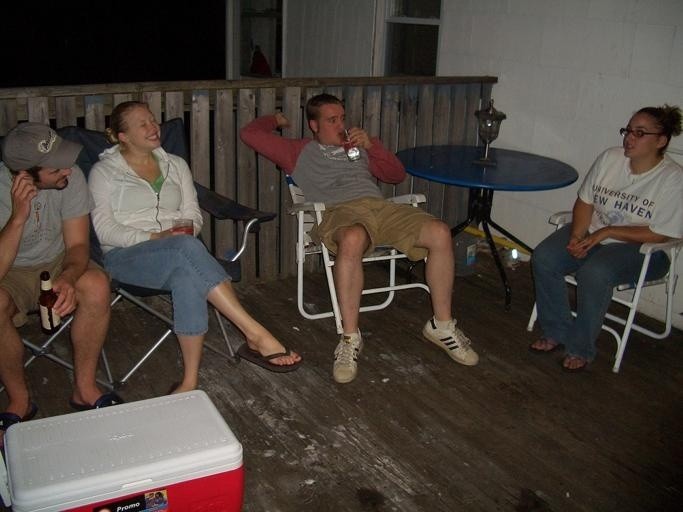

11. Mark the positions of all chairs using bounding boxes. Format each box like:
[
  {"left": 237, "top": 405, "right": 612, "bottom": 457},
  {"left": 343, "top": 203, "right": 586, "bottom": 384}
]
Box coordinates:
[
  {"left": 2, "top": 119, "right": 279, "bottom": 391},
  {"left": 285, "top": 171, "right": 433, "bottom": 336},
  {"left": 528, "top": 142, "right": 683, "bottom": 374}
]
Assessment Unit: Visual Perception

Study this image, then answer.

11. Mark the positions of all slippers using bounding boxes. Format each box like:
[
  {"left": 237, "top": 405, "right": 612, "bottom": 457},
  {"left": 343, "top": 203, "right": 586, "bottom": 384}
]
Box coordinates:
[
  {"left": 235, "top": 338, "right": 301, "bottom": 374},
  {"left": 165, "top": 380, "right": 182, "bottom": 396},
  {"left": 0, "top": 400, "right": 38, "bottom": 451},
  {"left": 68, "top": 393, "right": 123, "bottom": 411}
]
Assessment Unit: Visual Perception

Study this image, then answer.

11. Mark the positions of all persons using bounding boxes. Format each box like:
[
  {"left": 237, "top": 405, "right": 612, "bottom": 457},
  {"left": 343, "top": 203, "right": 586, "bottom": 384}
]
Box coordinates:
[
  {"left": 85, "top": 100, "right": 303, "bottom": 398},
  {"left": 525, "top": 100, "right": 683, "bottom": 373},
  {"left": 0, "top": 120, "right": 128, "bottom": 449},
  {"left": 238, "top": 92, "right": 481, "bottom": 384}
]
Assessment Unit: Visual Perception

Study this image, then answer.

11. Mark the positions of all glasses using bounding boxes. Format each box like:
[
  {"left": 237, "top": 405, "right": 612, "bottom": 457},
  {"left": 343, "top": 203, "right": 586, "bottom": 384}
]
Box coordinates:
[{"left": 619, "top": 126, "right": 661, "bottom": 137}]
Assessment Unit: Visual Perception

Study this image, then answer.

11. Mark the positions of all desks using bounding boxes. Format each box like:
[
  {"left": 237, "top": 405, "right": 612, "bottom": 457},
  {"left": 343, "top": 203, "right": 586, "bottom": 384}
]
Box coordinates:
[{"left": 399, "top": 144, "right": 579, "bottom": 310}]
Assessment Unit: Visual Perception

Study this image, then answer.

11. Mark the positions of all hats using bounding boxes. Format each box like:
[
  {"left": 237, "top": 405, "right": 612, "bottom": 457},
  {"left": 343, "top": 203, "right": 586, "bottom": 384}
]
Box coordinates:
[{"left": 2, "top": 120, "right": 83, "bottom": 171}]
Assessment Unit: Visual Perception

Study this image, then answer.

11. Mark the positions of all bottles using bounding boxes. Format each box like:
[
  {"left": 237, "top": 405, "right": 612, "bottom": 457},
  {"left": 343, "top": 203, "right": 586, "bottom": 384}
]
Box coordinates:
[
  {"left": 37, "top": 270, "right": 61, "bottom": 335},
  {"left": 341, "top": 126, "right": 362, "bottom": 162}
]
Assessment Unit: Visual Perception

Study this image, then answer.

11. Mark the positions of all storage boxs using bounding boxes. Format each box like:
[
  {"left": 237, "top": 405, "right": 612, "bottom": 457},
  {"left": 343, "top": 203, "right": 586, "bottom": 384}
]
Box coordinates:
[{"left": 0, "top": 390, "right": 244, "bottom": 511}]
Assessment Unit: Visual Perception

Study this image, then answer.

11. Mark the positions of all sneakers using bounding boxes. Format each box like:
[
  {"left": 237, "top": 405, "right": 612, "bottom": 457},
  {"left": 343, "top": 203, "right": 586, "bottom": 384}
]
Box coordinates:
[
  {"left": 333, "top": 325, "right": 362, "bottom": 384},
  {"left": 422, "top": 314, "right": 480, "bottom": 366}
]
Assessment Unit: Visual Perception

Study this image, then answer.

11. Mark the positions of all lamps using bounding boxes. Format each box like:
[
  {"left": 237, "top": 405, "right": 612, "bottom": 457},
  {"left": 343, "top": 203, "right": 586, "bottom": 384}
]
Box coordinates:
[{"left": 474, "top": 100, "right": 506, "bottom": 166}]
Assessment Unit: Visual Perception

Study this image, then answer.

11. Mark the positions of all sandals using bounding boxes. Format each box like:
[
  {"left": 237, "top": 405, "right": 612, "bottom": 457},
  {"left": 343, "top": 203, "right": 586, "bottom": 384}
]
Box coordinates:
[
  {"left": 561, "top": 352, "right": 588, "bottom": 371},
  {"left": 529, "top": 335, "right": 564, "bottom": 353}
]
